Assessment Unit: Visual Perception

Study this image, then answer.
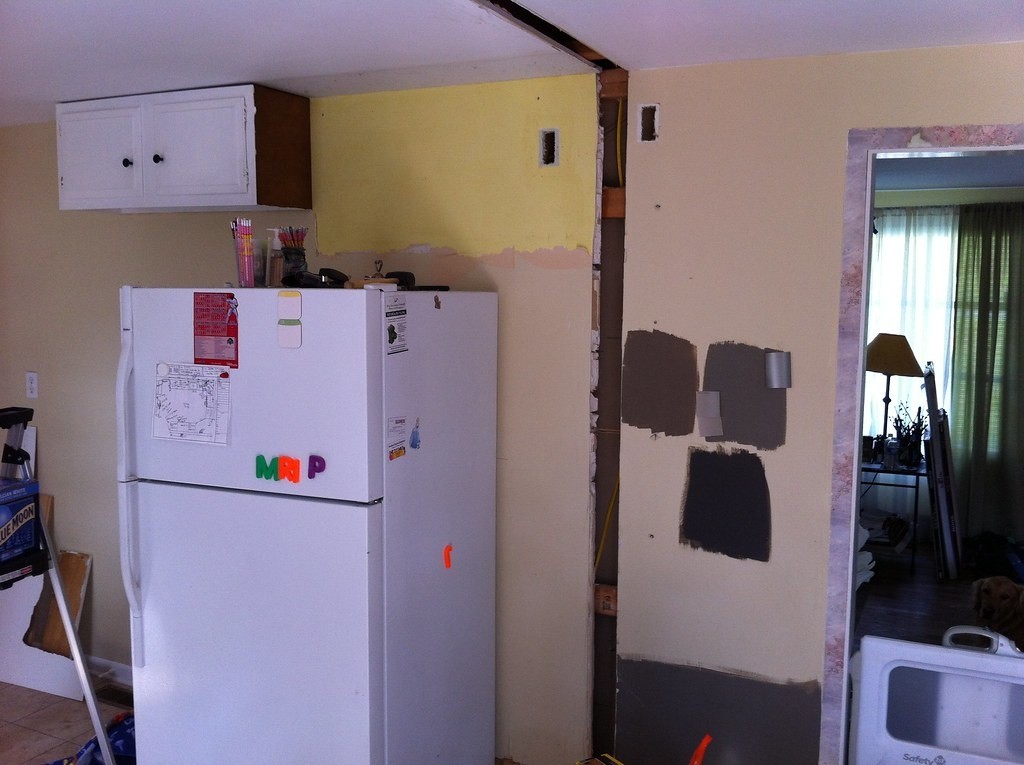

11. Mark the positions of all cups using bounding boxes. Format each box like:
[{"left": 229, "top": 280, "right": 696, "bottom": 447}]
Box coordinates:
[
  {"left": 235, "top": 239, "right": 265, "bottom": 288},
  {"left": 279, "top": 247, "right": 306, "bottom": 289}
]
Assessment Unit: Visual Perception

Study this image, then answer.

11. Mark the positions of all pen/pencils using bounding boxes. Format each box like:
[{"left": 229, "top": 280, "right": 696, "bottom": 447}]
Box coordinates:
[
  {"left": 231, "top": 217, "right": 256, "bottom": 287},
  {"left": 275, "top": 224, "right": 309, "bottom": 249}
]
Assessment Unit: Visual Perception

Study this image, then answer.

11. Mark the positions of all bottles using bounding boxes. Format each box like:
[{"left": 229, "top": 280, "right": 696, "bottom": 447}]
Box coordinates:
[
  {"left": 886, "top": 438, "right": 899, "bottom": 470},
  {"left": 884, "top": 434, "right": 894, "bottom": 468}
]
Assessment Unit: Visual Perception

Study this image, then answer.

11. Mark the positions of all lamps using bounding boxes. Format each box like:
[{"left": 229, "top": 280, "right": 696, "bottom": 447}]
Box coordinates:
[{"left": 865, "top": 333, "right": 925, "bottom": 461}]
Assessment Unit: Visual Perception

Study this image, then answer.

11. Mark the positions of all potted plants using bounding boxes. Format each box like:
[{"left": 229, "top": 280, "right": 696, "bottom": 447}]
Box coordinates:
[{"left": 890, "top": 393, "right": 928, "bottom": 465}]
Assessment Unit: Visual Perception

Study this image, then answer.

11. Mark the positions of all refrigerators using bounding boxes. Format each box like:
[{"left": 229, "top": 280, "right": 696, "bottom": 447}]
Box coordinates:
[{"left": 115, "top": 284, "right": 499, "bottom": 765}]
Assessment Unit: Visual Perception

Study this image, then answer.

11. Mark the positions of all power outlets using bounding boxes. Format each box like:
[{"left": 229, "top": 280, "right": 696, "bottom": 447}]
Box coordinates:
[{"left": 24, "top": 372, "right": 38, "bottom": 399}]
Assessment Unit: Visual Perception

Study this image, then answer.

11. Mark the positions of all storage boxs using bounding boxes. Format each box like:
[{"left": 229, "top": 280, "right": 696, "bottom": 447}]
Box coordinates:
[{"left": 0, "top": 477, "right": 39, "bottom": 560}]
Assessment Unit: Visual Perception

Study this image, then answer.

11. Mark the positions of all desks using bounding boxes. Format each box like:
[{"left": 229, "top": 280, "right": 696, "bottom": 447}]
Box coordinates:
[{"left": 861, "top": 449, "right": 930, "bottom": 576}]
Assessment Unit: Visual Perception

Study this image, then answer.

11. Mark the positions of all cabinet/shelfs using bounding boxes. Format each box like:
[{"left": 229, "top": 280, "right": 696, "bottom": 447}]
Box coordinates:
[{"left": 53, "top": 85, "right": 312, "bottom": 210}]
